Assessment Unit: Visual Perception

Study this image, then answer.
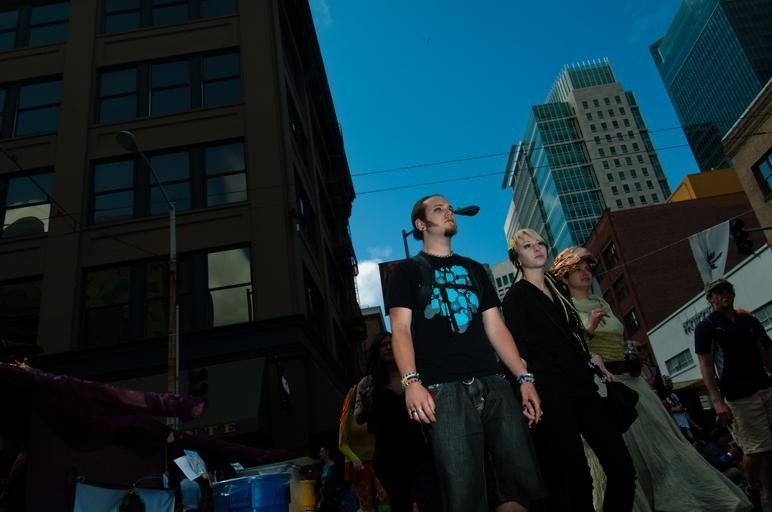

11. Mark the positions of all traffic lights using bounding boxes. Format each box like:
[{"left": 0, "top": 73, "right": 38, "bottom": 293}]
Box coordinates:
[{"left": 730, "top": 219, "right": 755, "bottom": 256}]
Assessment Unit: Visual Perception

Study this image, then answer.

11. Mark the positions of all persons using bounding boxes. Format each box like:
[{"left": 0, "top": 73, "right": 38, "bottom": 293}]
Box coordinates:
[
  {"left": 319, "top": 331, "right": 444, "bottom": 510},
  {"left": 502, "top": 229, "right": 638, "bottom": 511},
  {"left": 180, "top": 474, "right": 201, "bottom": 512},
  {"left": 633, "top": 278, "right": 771, "bottom": 511},
  {"left": 387, "top": 194, "right": 540, "bottom": 510},
  {"left": 549, "top": 246, "right": 754, "bottom": 510}
]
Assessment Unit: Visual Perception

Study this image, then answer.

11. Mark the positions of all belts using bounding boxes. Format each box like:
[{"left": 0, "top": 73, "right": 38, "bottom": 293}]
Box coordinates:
[{"left": 430, "top": 371, "right": 492, "bottom": 385}]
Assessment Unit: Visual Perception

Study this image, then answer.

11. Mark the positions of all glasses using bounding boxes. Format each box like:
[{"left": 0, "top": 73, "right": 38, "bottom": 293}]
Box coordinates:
[{"left": 710, "top": 287, "right": 731, "bottom": 296}]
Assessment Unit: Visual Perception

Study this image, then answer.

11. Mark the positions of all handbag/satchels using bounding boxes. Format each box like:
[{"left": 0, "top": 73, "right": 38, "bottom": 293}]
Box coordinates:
[
  {"left": 343, "top": 385, "right": 355, "bottom": 482},
  {"left": 586, "top": 361, "right": 639, "bottom": 435}
]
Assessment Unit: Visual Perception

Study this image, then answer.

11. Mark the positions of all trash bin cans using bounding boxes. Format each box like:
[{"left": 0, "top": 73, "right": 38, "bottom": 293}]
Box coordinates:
[{"left": 212, "top": 472, "right": 317, "bottom": 512}]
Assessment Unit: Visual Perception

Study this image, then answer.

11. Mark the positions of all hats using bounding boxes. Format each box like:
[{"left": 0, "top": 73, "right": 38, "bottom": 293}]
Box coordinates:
[
  {"left": 551, "top": 247, "right": 596, "bottom": 281},
  {"left": 705, "top": 278, "right": 733, "bottom": 298}
]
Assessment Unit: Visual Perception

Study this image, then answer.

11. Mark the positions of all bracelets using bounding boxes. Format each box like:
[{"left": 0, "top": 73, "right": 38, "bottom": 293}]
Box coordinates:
[{"left": 516, "top": 373, "right": 535, "bottom": 384}]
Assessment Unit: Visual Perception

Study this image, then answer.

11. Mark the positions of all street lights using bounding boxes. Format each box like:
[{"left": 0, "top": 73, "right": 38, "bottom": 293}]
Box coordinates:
[
  {"left": 401, "top": 204, "right": 481, "bottom": 260},
  {"left": 112, "top": 127, "right": 181, "bottom": 472}
]
